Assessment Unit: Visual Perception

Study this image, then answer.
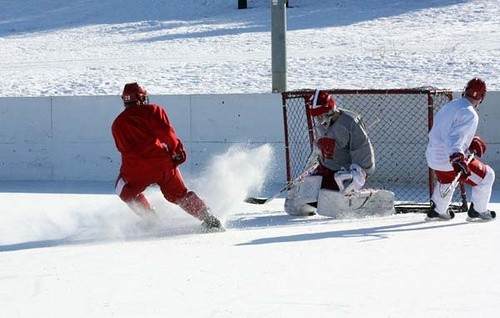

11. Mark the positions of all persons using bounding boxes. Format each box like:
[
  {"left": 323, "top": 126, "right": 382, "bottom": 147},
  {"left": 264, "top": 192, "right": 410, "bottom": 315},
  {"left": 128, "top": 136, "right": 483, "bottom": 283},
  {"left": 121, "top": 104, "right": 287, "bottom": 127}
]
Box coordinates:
[
  {"left": 284, "top": 89, "right": 375, "bottom": 217},
  {"left": 425, "top": 77, "right": 497, "bottom": 223},
  {"left": 111, "top": 83, "right": 226, "bottom": 234}
]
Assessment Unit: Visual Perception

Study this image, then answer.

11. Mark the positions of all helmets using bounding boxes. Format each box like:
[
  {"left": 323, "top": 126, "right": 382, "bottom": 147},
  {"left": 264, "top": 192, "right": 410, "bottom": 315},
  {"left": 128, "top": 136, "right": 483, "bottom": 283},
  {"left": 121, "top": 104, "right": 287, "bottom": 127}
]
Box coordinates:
[
  {"left": 305, "top": 90, "right": 334, "bottom": 115},
  {"left": 461, "top": 77, "right": 487, "bottom": 103},
  {"left": 122, "top": 82, "right": 149, "bottom": 104}
]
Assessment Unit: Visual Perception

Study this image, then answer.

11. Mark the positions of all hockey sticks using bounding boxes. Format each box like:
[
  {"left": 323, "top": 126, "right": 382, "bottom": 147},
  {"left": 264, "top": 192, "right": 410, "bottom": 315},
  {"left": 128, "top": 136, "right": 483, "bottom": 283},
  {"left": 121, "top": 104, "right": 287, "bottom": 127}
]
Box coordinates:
[
  {"left": 243, "top": 117, "right": 380, "bottom": 205},
  {"left": 439, "top": 150, "right": 476, "bottom": 198}
]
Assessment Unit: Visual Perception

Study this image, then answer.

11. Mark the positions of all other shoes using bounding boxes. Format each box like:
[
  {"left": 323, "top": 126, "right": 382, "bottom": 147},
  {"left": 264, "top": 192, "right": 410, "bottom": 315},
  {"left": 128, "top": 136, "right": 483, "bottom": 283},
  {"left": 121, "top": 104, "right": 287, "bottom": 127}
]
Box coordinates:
[
  {"left": 425, "top": 200, "right": 455, "bottom": 221},
  {"left": 201, "top": 215, "right": 222, "bottom": 229},
  {"left": 467, "top": 202, "right": 496, "bottom": 222}
]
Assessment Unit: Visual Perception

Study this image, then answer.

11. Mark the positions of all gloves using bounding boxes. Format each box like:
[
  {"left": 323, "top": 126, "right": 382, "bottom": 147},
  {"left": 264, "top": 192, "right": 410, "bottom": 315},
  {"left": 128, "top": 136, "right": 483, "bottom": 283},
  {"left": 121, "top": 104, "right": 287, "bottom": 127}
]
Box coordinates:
[
  {"left": 172, "top": 139, "right": 186, "bottom": 165},
  {"left": 468, "top": 135, "right": 487, "bottom": 158},
  {"left": 450, "top": 152, "right": 471, "bottom": 179}
]
